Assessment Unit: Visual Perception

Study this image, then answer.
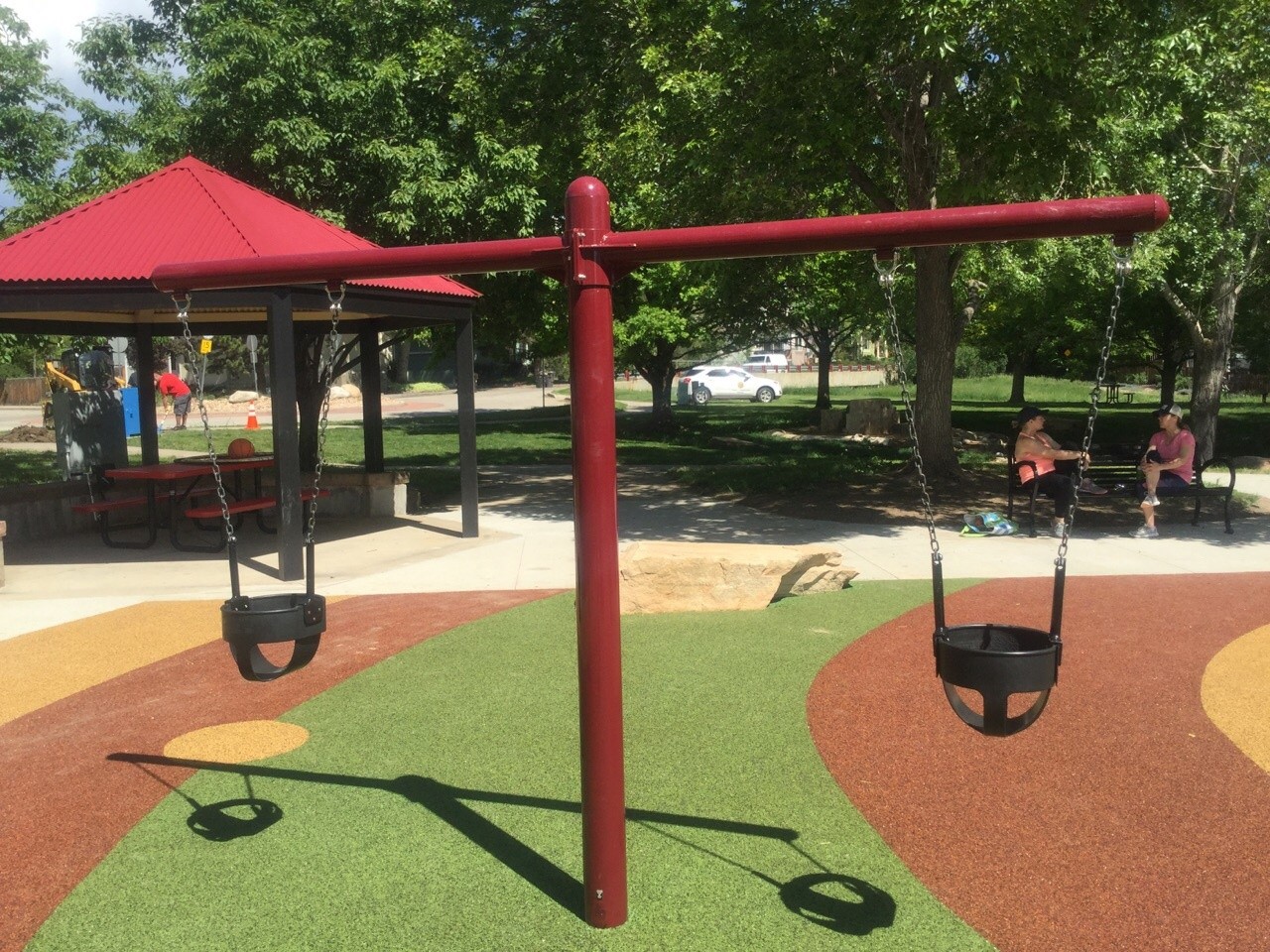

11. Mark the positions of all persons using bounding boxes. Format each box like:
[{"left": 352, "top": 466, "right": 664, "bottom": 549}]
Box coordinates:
[
  {"left": 153, "top": 371, "right": 192, "bottom": 431},
  {"left": 127, "top": 372, "right": 138, "bottom": 388},
  {"left": 1128, "top": 403, "right": 1195, "bottom": 536},
  {"left": 1015, "top": 407, "right": 1107, "bottom": 538}
]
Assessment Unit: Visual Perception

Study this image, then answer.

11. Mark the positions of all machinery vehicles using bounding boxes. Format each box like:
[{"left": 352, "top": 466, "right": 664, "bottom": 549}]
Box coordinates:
[{"left": 40, "top": 345, "right": 130, "bottom": 431}]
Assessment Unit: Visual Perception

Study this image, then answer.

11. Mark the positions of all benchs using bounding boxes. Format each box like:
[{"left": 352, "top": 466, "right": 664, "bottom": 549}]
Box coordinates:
[
  {"left": 1124, "top": 392, "right": 1135, "bottom": 405},
  {"left": 1004, "top": 444, "right": 1236, "bottom": 535},
  {"left": 71, "top": 487, "right": 218, "bottom": 550},
  {"left": 186, "top": 489, "right": 331, "bottom": 553},
  {"left": 1089, "top": 392, "right": 1099, "bottom": 404}
]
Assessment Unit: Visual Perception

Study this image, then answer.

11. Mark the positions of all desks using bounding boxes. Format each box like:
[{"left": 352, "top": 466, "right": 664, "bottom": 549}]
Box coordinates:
[
  {"left": 1099, "top": 384, "right": 1125, "bottom": 404},
  {"left": 105, "top": 452, "right": 275, "bottom": 551}
]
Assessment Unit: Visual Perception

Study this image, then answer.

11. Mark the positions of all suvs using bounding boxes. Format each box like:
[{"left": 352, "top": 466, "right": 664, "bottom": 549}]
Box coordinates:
[{"left": 676, "top": 365, "right": 783, "bottom": 405}]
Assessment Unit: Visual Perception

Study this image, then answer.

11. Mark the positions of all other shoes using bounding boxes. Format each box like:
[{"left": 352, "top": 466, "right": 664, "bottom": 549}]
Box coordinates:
[{"left": 173, "top": 426, "right": 187, "bottom": 431}]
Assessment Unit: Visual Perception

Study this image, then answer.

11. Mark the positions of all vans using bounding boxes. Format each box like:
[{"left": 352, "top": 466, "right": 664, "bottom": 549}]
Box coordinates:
[{"left": 742, "top": 353, "right": 788, "bottom": 373}]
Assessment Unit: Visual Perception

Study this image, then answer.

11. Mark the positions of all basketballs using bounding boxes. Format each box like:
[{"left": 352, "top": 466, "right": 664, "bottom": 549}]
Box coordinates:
[{"left": 227, "top": 438, "right": 255, "bottom": 459}]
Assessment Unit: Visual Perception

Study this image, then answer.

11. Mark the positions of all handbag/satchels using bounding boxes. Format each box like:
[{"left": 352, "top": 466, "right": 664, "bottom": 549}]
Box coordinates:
[{"left": 960, "top": 512, "right": 1020, "bottom": 537}]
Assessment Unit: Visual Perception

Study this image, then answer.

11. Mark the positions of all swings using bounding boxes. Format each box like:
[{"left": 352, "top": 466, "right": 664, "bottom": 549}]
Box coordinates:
[
  {"left": 876, "top": 240, "right": 1135, "bottom": 737},
  {"left": 172, "top": 288, "right": 350, "bottom": 683}
]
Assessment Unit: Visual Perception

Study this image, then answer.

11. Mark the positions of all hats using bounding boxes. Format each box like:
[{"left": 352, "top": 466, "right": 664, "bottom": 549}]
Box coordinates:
[
  {"left": 1018, "top": 407, "right": 1050, "bottom": 425},
  {"left": 1152, "top": 404, "right": 1183, "bottom": 418}
]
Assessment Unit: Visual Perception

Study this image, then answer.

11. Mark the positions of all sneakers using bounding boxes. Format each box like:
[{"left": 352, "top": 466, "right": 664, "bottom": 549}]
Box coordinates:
[
  {"left": 1140, "top": 492, "right": 1162, "bottom": 509},
  {"left": 1050, "top": 517, "right": 1064, "bottom": 538},
  {"left": 1127, "top": 523, "right": 1160, "bottom": 538},
  {"left": 1079, "top": 481, "right": 1108, "bottom": 495}
]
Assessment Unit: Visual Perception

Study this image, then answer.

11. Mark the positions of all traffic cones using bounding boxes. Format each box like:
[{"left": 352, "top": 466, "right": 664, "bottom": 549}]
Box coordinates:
[{"left": 241, "top": 399, "right": 262, "bottom": 430}]
